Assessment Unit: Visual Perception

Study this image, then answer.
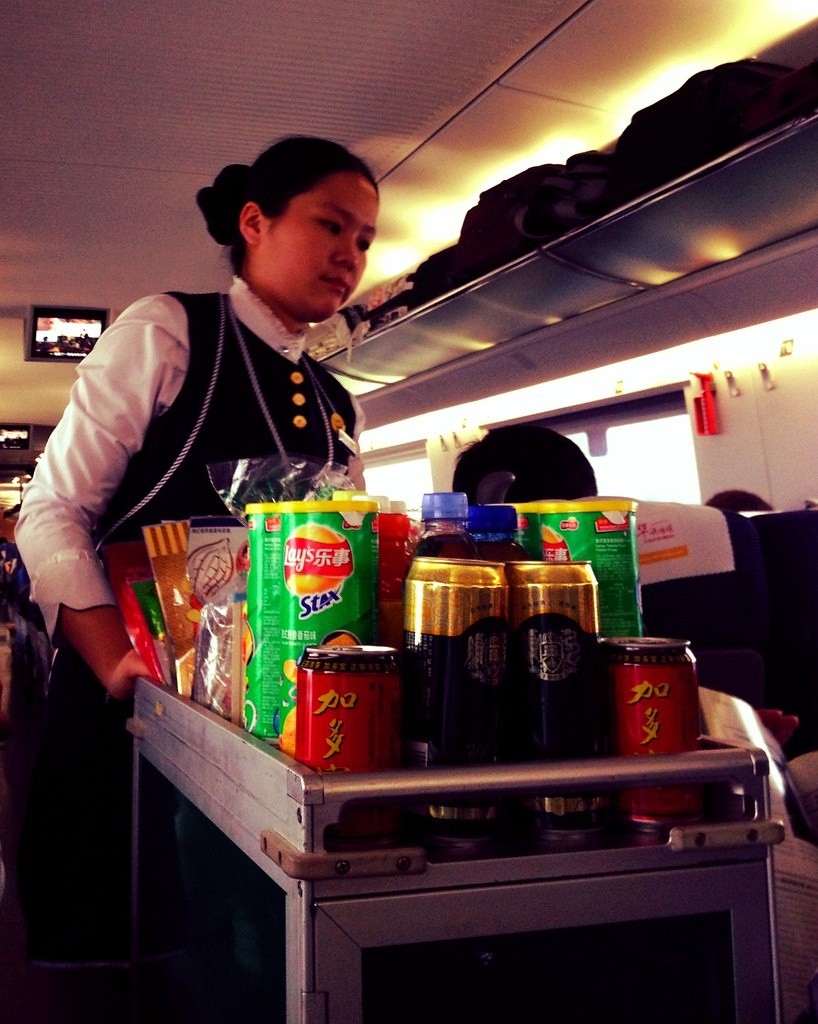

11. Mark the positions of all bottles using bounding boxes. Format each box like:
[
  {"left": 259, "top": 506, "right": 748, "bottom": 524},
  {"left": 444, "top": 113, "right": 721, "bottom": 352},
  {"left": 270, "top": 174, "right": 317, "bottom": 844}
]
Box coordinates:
[{"left": 315, "top": 484, "right": 530, "bottom": 663}]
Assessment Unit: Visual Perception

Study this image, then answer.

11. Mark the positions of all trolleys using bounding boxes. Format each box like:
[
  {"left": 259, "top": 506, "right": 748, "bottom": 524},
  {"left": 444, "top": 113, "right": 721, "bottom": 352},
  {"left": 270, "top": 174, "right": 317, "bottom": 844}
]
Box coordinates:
[{"left": 128, "top": 665, "right": 784, "bottom": 1024}]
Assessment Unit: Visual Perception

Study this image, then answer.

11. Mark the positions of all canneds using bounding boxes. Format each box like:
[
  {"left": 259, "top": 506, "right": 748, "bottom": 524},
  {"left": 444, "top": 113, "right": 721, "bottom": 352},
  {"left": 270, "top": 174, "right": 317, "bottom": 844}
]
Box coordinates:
[{"left": 297, "top": 558, "right": 703, "bottom": 840}]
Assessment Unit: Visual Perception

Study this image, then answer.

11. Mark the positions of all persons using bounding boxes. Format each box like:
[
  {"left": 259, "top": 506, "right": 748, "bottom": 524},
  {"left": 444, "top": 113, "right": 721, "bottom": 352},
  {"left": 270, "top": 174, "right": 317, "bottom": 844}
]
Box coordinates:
[
  {"left": 702, "top": 489, "right": 774, "bottom": 518},
  {"left": 36, "top": 317, "right": 55, "bottom": 337},
  {"left": 451, "top": 423, "right": 603, "bottom": 509},
  {"left": 746, "top": 698, "right": 818, "bottom": 829},
  {"left": 7, "top": 129, "right": 387, "bottom": 1022}
]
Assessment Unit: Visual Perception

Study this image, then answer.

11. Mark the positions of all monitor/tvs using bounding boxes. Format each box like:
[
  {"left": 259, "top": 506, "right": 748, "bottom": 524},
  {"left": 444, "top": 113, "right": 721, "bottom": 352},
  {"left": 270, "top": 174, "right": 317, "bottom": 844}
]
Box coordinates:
[
  {"left": 1, "top": 423, "right": 33, "bottom": 453},
  {"left": 25, "top": 304, "right": 109, "bottom": 363}
]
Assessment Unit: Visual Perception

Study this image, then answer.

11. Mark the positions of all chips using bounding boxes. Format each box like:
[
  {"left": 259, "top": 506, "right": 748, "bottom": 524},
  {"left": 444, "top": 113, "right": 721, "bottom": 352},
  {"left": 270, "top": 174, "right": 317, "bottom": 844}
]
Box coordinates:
[
  {"left": 494, "top": 497, "right": 645, "bottom": 642},
  {"left": 247, "top": 500, "right": 381, "bottom": 752}
]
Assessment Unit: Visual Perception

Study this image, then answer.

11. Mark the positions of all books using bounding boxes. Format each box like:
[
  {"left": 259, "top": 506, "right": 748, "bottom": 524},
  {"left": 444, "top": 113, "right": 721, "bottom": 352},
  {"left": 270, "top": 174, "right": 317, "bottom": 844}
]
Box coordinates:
[{"left": 695, "top": 684, "right": 815, "bottom": 858}]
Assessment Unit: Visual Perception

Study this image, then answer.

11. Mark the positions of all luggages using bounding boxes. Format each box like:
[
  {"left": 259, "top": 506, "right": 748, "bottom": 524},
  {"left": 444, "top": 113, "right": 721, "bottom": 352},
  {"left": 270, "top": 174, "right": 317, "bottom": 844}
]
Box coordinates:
[{"left": 306, "top": 60, "right": 817, "bottom": 363}]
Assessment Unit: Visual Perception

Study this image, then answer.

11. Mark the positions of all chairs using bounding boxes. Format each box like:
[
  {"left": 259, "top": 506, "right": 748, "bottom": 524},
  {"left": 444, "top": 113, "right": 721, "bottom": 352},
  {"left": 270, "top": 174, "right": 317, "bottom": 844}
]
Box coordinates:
[
  {"left": 738, "top": 505, "right": 818, "bottom": 762},
  {"left": 578, "top": 495, "right": 780, "bottom": 708}
]
